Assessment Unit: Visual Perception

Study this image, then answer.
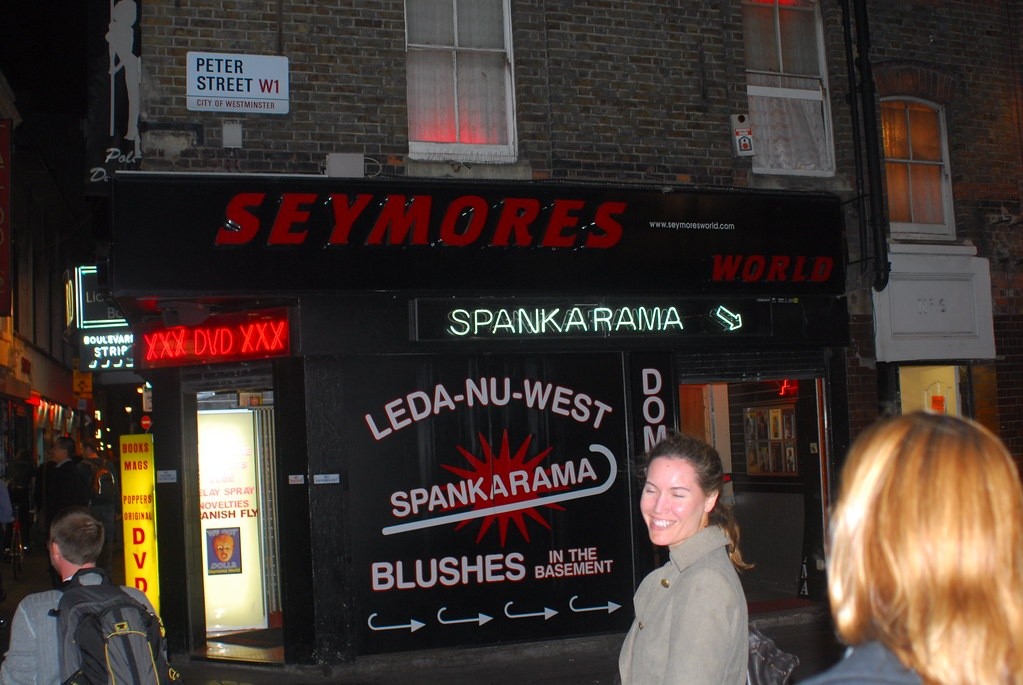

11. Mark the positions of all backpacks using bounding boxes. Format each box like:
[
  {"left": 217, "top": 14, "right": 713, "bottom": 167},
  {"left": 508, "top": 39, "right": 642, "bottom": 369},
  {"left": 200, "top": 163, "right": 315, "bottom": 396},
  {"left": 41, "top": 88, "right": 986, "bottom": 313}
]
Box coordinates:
[{"left": 56, "top": 568, "right": 184, "bottom": 685}]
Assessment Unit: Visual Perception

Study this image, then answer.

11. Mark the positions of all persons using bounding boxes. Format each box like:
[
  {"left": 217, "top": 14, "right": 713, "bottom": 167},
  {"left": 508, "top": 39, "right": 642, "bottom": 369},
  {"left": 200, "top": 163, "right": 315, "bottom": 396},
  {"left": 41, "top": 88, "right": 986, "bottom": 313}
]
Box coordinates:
[
  {"left": 0, "top": 437, "right": 117, "bottom": 629},
  {"left": 795, "top": 410, "right": 1023, "bottom": 685},
  {"left": 618, "top": 436, "right": 749, "bottom": 685},
  {"left": 0, "top": 506, "right": 167, "bottom": 685}
]
